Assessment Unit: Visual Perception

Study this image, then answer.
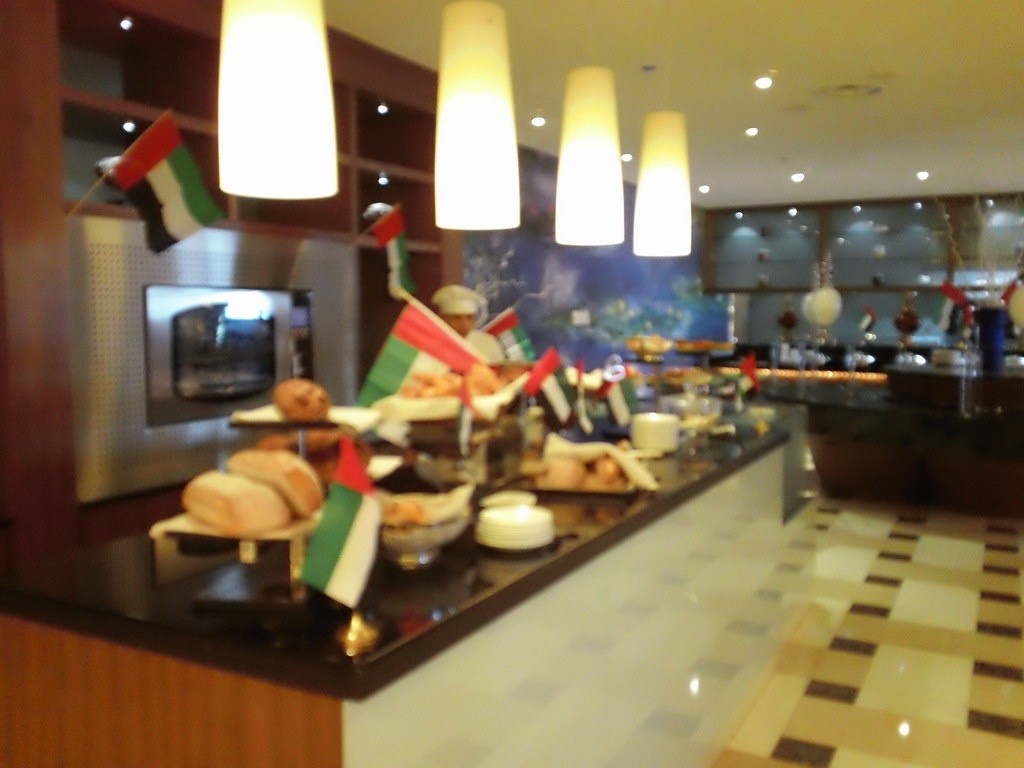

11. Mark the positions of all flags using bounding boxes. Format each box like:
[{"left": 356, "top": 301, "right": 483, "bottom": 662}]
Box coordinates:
[
  {"left": 604, "top": 378, "right": 638, "bottom": 427},
  {"left": 857, "top": 308, "right": 875, "bottom": 331},
  {"left": 357, "top": 302, "right": 474, "bottom": 408},
  {"left": 734, "top": 355, "right": 761, "bottom": 412},
  {"left": 524, "top": 348, "right": 578, "bottom": 425},
  {"left": 105, "top": 115, "right": 225, "bottom": 253},
  {"left": 373, "top": 210, "right": 417, "bottom": 298},
  {"left": 930, "top": 282, "right": 968, "bottom": 335},
  {"left": 487, "top": 312, "right": 536, "bottom": 361},
  {"left": 297, "top": 432, "right": 382, "bottom": 608}
]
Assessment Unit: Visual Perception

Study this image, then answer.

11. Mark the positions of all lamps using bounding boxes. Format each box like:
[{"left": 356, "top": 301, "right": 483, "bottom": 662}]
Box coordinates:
[
  {"left": 802, "top": 260, "right": 843, "bottom": 333},
  {"left": 555, "top": 67, "right": 625, "bottom": 246},
  {"left": 217, "top": 0, "right": 338, "bottom": 200},
  {"left": 434, "top": 1, "right": 520, "bottom": 231},
  {"left": 633, "top": 111, "right": 691, "bottom": 256}
]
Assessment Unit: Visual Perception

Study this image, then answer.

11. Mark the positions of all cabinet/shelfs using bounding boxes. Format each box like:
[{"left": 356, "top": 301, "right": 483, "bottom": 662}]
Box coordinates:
[{"left": 1, "top": 0, "right": 460, "bottom": 565}]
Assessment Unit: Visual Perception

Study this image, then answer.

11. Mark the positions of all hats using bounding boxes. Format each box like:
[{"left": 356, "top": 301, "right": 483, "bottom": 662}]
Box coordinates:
[{"left": 432, "top": 286, "right": 484, "bottom": 316}]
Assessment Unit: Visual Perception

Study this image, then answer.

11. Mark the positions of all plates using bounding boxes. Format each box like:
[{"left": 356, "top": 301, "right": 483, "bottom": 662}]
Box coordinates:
[
  {"left": 478, "top": 490, "right": 537, "bottom": 507},
  {"left": 474, "top": 505, "right": 554, "bottom": 549},
  {"left": 631, "top": 412, "right": 679, "bottom": 452}
]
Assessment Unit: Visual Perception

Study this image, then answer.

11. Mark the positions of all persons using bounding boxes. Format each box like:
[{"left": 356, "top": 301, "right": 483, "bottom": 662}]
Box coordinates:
[{"left": 431, "top": 283, "right": 506, "bottom": 364}]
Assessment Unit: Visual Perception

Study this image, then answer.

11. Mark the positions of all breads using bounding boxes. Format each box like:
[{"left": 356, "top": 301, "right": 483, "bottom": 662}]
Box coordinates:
[
  {"left": 547, "top": 455, "right": 620, "bottom": 488},
  {"left": 399, "top": 362, "right": 500, "bottom": 398},
  {"left": 180, "top": 377, "right": 345, "bottom": 537}
]
